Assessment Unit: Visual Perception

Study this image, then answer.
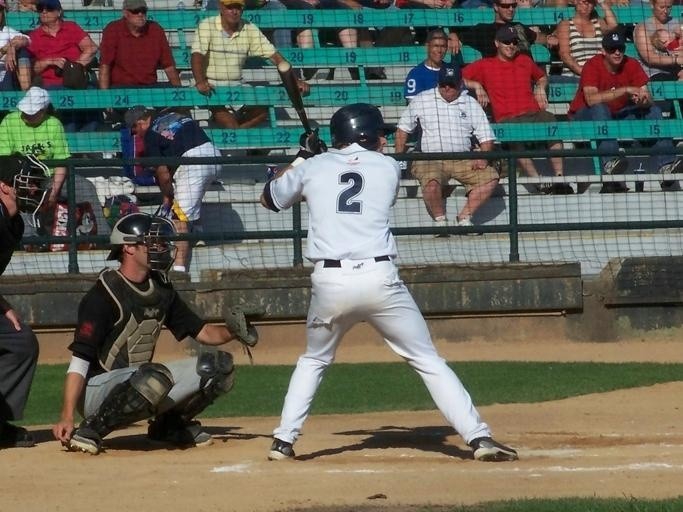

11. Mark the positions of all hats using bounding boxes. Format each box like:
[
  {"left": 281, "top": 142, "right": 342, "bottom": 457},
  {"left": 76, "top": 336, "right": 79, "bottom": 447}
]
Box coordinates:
[
  {"left": 495, "top": 25, "right": 521, "bottom": 42},
  {"left": 16, "top": 87, "right": 50, "bottom": 115},
  {"left": 123, "top": 106, "right": 147, "bottom": 137},
  {"left": 124, "top": 1, "right": 147, "bottom": 10},
  {"left": 425, "top": 29, "right": 451, "bottom": 42},
  {"left": 439, "top": 63, "right": 460, "bottom": 83},
  {"left": 601, "top": 31, "right": 626, "bottom": 49},
  {"left": 220, "top": 1, "right": 246, "bottom": 8}
]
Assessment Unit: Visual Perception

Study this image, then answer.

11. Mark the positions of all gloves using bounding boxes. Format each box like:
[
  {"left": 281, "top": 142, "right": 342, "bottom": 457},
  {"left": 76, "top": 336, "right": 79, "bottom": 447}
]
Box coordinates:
[{"left": 298, "top": 128, "right": 321, "bottom": 160}]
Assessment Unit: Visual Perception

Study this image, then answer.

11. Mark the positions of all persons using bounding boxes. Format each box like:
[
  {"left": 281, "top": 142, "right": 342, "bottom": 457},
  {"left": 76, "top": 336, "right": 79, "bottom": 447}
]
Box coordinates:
[
  {"left": 0, "top": 0, "right": 182, "bottom": 130},
  {"left": 125, "top": 106, "right": 224, "bottom": 275},
  {"left": 0, "top": 87, "right": 71, "bottom": 252},
  {"left": 190, "top": 1, "right": 311, "bottom": 129},
  {"left": 52, "top": 213, "right": 236, "bottom": 454},
  {"left": 1, "top": 152, "right": 51, "bottom": 447},
  {"left": 260, "top": 104, "right": 517, "bottom": 460},
  {"left": 287, "top": 1, "right": 682, "bottom": 234}
]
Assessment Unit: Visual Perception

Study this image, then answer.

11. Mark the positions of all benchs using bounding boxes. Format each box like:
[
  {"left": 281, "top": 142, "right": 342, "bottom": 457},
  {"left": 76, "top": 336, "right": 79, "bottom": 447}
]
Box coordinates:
[{"left": 0, "top": 1, "right": 682, "bottom": 199}]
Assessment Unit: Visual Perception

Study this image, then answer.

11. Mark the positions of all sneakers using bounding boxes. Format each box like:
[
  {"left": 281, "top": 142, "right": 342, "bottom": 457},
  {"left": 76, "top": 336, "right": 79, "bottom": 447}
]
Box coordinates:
[
  {"left": 536, "top": 182, "right": 575, "bottom": 194},
  {"left": 430, "top": 216, "right": 451, "bottom": 238},
  {"left": 453, "top": 216, "right": 483, "bottom": 236},
  {"left": 144, "top": 418, "right": 212, "bottom": 448},
  {"left": 0, "top": 421, "right": 36, "bottom": 448},
  {"left": 62, "top": 427, "right": 98, "bottom": 456},
  {"left": 268, "top": 438, "right": 294, "bottom": 462},
  {"left": 656, "top": 160, "right": 682, "bottom": 184},
  {"left": 603, "top": 157, "right": 626, "bottom": 174},
  {"left": 469, "top": 435, "right": 520, "bottom": 462}
]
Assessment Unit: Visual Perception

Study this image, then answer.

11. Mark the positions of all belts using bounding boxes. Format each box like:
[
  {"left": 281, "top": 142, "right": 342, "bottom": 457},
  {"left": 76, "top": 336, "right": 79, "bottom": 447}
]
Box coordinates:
[{"left": 323, "top": 255, "right": 389, "bottom": 267}]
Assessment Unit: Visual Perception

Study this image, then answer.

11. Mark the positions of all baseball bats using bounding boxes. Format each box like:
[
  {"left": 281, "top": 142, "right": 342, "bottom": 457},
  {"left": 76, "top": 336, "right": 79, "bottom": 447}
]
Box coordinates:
[{"left": 276, "top": 61, "right": 325, "bottom": 153}]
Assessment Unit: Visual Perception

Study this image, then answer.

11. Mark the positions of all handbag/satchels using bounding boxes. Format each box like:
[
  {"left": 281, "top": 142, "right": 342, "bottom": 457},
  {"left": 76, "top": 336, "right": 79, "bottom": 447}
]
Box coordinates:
[
  {"left": 48, "top": 204, "right": 97, "bottom": 250},
  {"left": 63, "top": 61, "right": 89, "bottom": 89}
]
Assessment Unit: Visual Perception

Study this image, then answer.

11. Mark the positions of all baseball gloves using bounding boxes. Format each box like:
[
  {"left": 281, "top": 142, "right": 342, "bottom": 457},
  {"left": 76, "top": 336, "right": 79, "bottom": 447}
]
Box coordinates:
[{"left": 224, "top": 304, "right": 266, "bottom": 347}]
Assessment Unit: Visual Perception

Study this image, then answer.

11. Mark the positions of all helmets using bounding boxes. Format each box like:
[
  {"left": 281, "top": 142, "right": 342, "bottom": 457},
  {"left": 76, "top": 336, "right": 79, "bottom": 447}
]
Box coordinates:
[
  {"left": 106, "top": 213, "right": 179, "bottom": 285},
  {"left": 330, "top": 103, "right": 398, "bottom": 147},
  {"left": 1, "top": 152, "right": 52, "bottom": 231}
]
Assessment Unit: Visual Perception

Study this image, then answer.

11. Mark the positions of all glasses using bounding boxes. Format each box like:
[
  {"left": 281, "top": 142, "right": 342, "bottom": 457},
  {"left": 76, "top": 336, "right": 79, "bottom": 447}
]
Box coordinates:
[
  {"left": 501, "top": 39, "right": 519, "bottom": 45},
  {"left": 604, "top": 45, "right": 626, "bottom": 53},
  {"left": 130, "top": 9, "right": 146, "bottom": 14},
  {"left": 498, "top": 4, "right": 518, "bottom": 9},
  {"left": 438, "top": 82, "right": 456, "bottom": 89},
  {"left": 36, "top": 2, "right": 56, "bottom": 13}
]
